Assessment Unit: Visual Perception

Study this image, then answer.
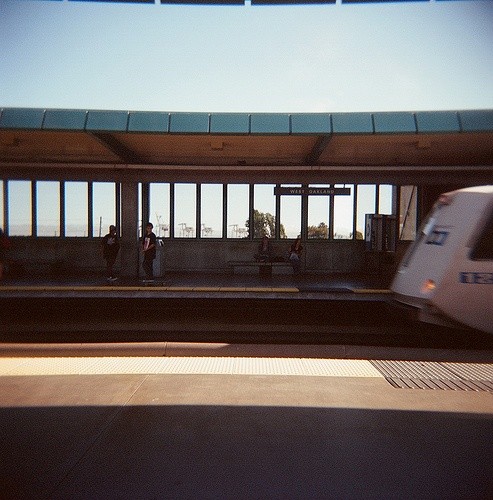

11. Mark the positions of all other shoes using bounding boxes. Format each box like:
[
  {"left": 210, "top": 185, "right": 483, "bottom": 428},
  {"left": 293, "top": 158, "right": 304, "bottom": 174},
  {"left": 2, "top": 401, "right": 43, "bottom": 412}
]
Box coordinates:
[
  {"left": 142, "top": 277, "right": 154, "bottom": 282},
  {"left": 107, "top": 276, "right": 118, "bottom": 280}
]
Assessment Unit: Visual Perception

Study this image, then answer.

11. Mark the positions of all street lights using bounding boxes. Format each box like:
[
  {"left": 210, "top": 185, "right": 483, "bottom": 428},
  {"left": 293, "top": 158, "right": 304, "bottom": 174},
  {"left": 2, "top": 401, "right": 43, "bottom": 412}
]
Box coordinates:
[{"left": 229, "top": 224, "right": 238, "bottom": 237}]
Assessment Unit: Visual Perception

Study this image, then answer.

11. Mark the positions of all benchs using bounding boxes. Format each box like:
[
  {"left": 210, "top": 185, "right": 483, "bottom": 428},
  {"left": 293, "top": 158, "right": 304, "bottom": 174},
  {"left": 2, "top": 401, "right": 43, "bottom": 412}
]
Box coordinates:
[{"left": 228, "top": 261, "right": 291, "bottom": 277}]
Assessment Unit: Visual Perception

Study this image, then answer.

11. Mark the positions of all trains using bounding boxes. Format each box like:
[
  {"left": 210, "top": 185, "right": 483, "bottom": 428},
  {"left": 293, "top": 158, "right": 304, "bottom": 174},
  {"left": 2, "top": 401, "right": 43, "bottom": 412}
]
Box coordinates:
[{"left": 387, "top": 184, "right": 492, "bottom": 343}]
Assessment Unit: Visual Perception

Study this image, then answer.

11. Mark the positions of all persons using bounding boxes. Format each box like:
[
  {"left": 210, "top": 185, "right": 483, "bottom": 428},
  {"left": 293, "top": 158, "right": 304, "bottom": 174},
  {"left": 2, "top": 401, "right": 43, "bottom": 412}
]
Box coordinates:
[
  {"left": 101, "top": 225, "right": 120, "bottom": 281},
  {"left": 141, "top": 222, "right": 157, "bottom": 282},
  {"left": 286, "top": 238, "right": 304, "bottom": 279},
  {"left": 257, "top": 237, "right": 273, "bottom": 283}
]
